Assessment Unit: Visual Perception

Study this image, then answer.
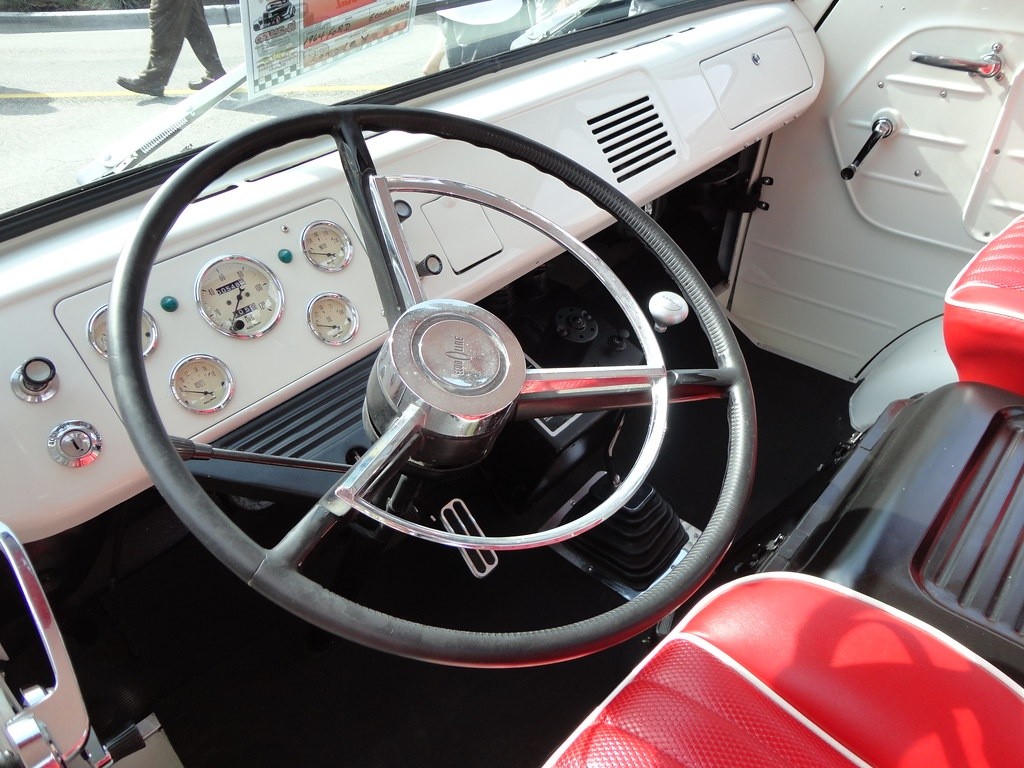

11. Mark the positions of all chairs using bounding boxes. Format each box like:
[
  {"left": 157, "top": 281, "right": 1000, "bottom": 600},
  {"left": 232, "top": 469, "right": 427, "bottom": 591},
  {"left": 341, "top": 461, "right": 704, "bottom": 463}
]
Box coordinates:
[
  {"left": 541, "top": 570, "right": 1024, "bottom": 768},
  {"left": 943, "top": 213, "right": 1024, "bottom": 397}
]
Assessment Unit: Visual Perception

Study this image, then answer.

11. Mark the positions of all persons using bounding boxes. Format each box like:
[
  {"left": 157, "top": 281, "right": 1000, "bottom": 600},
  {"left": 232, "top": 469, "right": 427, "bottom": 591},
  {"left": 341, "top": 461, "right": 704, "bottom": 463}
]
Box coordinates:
[
  {"left": 437, "top": 0, "right": 533, "bottom": 69},
  {"left": 116, "top": 0, "right": 226, "bottom": 97}
]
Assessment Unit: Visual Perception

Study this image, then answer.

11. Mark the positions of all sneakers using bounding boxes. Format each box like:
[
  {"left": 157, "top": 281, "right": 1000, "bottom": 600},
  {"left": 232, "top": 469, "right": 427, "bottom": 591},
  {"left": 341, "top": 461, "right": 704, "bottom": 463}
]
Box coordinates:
[
  {"left": 116, "top": 76, "right": 165, "bottom": 96},
  {"left": 189, "top": 78, "right": 212, "bottom": 91}
]
